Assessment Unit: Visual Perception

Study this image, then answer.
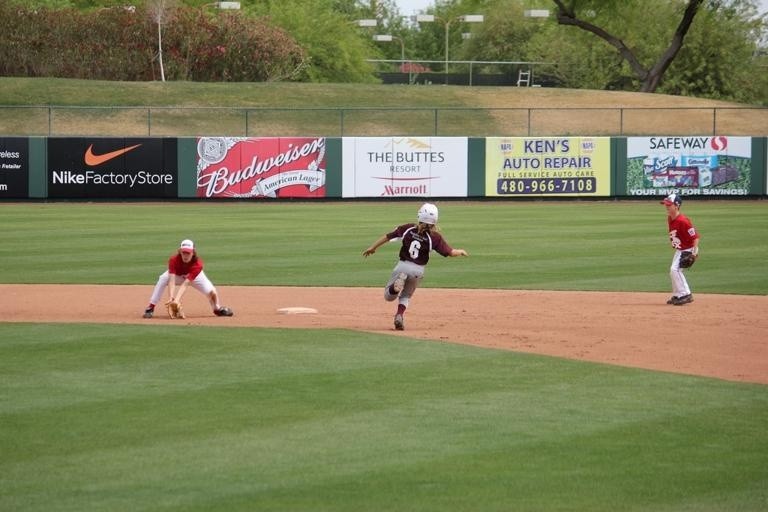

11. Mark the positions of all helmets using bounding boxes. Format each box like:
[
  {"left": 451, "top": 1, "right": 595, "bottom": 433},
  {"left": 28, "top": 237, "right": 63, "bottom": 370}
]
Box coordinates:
[{"left": 418, "top": 204, "right": 438, "bottom": 225}]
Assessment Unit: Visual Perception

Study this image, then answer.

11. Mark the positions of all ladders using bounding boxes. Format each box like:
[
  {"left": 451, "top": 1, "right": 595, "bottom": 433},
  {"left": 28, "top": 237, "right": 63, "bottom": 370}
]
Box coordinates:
[{"left": 517, "top": 69, "right": 531, "bottom": 87}]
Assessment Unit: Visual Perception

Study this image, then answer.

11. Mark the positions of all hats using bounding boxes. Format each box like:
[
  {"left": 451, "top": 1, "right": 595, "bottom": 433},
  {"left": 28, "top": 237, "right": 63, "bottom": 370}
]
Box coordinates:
[
  {"left": 661, "top": 194, "right": 681, "bottom": 205},
  {"left": 180, "top": 239, "right": 194, "bottom": 252}
]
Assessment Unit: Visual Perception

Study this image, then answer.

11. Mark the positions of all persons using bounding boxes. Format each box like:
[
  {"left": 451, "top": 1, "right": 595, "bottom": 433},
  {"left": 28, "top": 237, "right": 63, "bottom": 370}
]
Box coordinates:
[
  {"left": 659, "top": 193, "right": 700, "bottom": 305},
  {"left": 143, "top": 238, "right": 233, "bottom": 318},
  {"left": 362, "top": 203, "right": 467, "bottom": 330}
]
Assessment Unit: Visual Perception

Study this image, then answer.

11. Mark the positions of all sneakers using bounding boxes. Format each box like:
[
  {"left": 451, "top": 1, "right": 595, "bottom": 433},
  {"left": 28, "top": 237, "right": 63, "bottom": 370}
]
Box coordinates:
[
  {"left": 394, "top": 314, "right": 404, "bottom": 330},
  {"left": 214, "top": 307, "right": 233, "bottom": 316},
  {"left": 667, "top": 294, "right": 693, "bottom": 305},
  {"left": 393, "top": 274, "right": 407, "bottom": 292},
  {"left": 143, "top": 307, "right": 154, "bottom": 317}
]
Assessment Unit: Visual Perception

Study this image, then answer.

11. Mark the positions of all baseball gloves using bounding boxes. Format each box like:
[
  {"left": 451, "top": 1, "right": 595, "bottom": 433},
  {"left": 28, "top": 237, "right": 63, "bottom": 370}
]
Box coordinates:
[
  {"left": 166, "top": 299, "right": 185, "bottom": 319},
  {"left": 678, "top": 250, "right": 696, "bottom": 269}
]
